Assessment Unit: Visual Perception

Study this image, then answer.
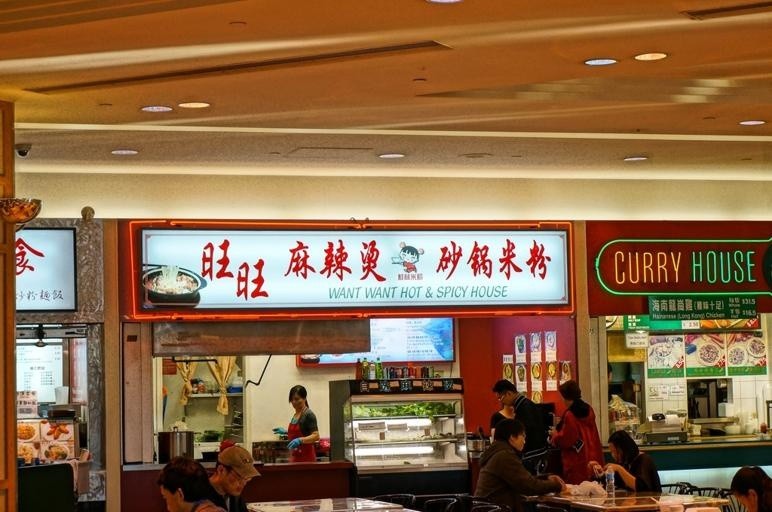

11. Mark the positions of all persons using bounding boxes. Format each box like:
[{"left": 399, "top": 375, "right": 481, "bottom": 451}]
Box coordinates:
[
  {"left": 489, "top": 403, "right": 518, "bottom": 441},
  {"left": 474, "top": 417, "right": 566, "bottom": 512},
  {"left": 588, "top": 430, "right": 663, "bottom": 492},
  {"left": 208, "top": 445, "right": 261, "bottom": 511},
  {"left": 491, "top": 379, "right": 549, "bottom": 475},
  {"left": 272, "top": 385, "right": 320, "bottom": 462},
  {"left": 547, "top": 379, "right": 606, "bottom": 486},
  {"left": 731, "top": 466, "right": 772, "bottom": 512},
  {"left": 157, "top": 455, "right": 225, "bottom": 511},
  {"left": 608, "top": 363, "right": 612, "bottom": 403}
]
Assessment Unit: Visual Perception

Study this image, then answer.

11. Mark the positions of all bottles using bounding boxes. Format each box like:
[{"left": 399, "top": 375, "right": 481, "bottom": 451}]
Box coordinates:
[
  {"left": 760, "top": 422, "right": 767, "bottom": 434},
  {"left": 195, "top": 432, "right": 200, "bottom": 443},
  {"left": 355, "top": 356, "right": 382, "bottom": 380},
  {"left": 605, "top": 465, "right": 615, "bottom": 495},
  {"left": 752, "top": 412, "right": 759, "bottom": 434}
]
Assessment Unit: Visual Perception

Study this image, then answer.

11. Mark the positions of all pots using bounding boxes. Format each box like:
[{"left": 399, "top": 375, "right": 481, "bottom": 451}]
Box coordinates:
[
  {"left": 157, "top": 427, "right": 195, "bottom": 465},
  {"left": 466, "top": 426, "right": 490, "bottom": 452}
]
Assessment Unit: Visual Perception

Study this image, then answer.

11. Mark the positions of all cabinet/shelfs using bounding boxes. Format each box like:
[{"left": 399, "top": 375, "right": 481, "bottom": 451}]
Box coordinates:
[
  {"left": 187, "top": 391, "right": 244, "bottom": 460},
  {"left": 343, "top": 398, "right": 468, "bottom": 470}
]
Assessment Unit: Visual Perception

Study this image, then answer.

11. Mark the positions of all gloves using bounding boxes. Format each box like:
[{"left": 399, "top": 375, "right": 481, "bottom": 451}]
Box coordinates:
[
  {"left": 287, "top": 438, "right": 302, "bottom": 450},
  {"left": 272, "top": 427, "right": 287, "bottom": 434}
]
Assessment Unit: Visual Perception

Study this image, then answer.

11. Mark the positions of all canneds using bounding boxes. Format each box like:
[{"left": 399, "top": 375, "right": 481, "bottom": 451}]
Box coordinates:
[{"left": 383, "top": 366, "right": 434, "bottom": 378}]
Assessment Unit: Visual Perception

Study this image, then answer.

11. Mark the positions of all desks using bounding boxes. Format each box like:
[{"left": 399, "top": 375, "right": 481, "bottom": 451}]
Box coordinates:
[{"left": 245, "top": 496, "right": 422, "bottom": 512}]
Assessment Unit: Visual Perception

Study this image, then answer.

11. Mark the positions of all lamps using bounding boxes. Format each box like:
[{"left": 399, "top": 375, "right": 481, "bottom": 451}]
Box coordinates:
[{"left": 1, "top": 196, "right": 43, "bottom": 232}]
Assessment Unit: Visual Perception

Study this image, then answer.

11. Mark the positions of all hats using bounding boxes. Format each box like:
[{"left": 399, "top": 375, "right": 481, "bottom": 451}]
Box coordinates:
[{"left": 218, "top": 446, "right": 262, "bottom": 480}]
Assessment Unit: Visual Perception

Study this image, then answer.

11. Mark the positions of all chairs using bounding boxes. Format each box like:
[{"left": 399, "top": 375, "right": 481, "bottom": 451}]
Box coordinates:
[{"left": 371, "top": 481, "right": 746, "bottom": 512}]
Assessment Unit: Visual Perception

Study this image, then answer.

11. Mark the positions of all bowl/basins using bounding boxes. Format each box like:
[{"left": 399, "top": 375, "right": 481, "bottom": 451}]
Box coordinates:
[{"left": 142, "top": 266, "right": 208, "bottom": 304}]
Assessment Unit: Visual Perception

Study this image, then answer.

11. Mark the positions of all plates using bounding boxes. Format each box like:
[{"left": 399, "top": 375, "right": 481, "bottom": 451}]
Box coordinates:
[
  {"left": 504, "top": 333, "right": 542, "bottom": 403},
  {"left": 43, "top": 444, "right": 70, "bottom": 460},
  {"left": 43, "top": 426, "right": 72, "bottom": 441},
  {"left": 17, "top": 423, "right": 38, "bottom": 442},
  {"left": 562, "top": 362, "right": 570, "bottom": 374},
  {"left": 694, "top": 338, "right": 766, "bottom": 367},
  {"left": 655, "top": 344, "right": 671, "bottom": 357},
  {"left": 548, "top": 363, "right": 556, "bottom": 378},
  {"left": 547, "top": 334, "right": 555, "bottom": 346},
  {"left": 147, "top": 291, "right": 201, "bottom": 308},
  {"left": 20, "top": 443, "right": 38, "bottom": 461}
]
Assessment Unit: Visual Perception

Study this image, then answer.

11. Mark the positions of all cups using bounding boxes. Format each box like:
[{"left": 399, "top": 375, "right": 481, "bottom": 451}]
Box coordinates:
[
  {"left": 670, "top": 505, "right": 683, "bottom": 512},
  {"left": 745, "top": 423, "right": 754, "bottom": 434},
  {"left": 55, "top": 386, "right": 69, "bottom": 405}
]
